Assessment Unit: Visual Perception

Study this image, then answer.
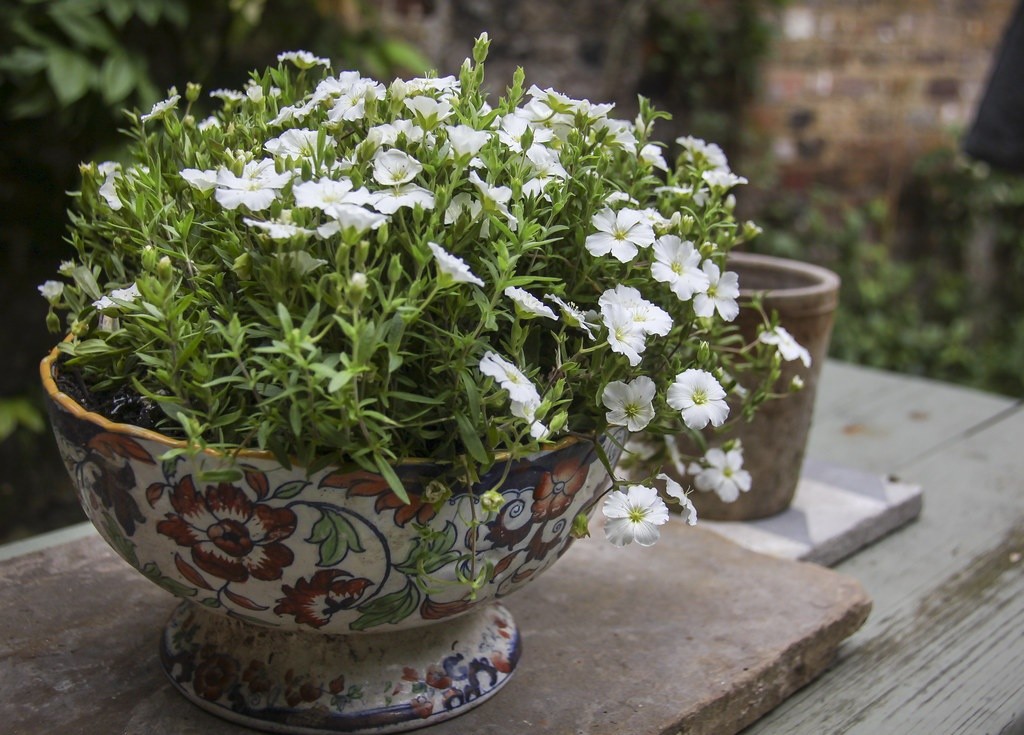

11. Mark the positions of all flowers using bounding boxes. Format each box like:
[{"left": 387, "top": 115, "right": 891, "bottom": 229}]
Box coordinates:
[{"left": 36, "top": 33, "right": 815, "bottom": 607}]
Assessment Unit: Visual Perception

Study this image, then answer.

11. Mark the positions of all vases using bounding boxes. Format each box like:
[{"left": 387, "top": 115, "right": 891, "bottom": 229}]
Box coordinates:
[
  {"left": 632, "top": 255, "right": 841, "bottom": 524},
  {"left": 41, "top": 333, "right": 630, "bottom": 735}
]
